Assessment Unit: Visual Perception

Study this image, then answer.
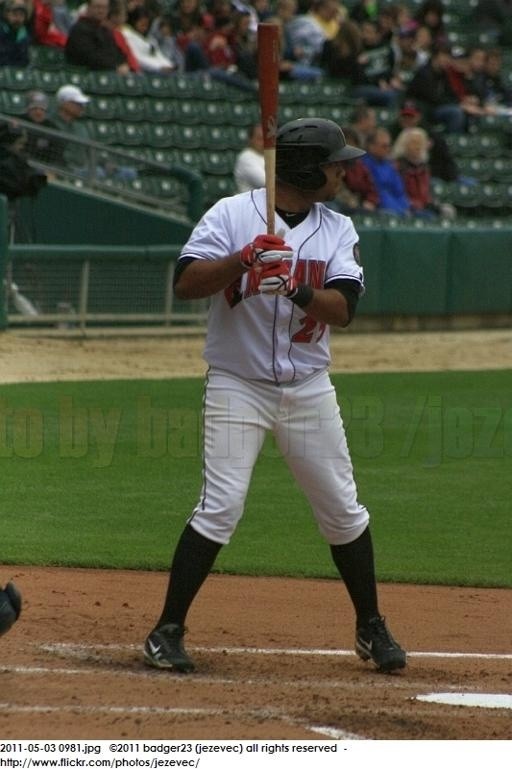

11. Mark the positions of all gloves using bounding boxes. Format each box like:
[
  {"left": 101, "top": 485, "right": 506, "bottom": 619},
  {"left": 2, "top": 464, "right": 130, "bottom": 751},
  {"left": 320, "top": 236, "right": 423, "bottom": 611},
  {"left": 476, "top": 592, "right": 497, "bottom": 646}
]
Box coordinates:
[
  {"left": 241, "top": 228, "right": 293, "bottom": 268},
  {"left": 254, "top": 262, "right": 299, "bottom": 298}
]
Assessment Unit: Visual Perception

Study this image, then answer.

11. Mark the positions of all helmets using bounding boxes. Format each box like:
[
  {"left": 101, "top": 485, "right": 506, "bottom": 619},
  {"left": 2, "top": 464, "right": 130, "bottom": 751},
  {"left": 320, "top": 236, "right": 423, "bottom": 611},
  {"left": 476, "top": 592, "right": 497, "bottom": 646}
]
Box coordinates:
[{"left": 274, "top": 116, "right": 368, "bottom": 192}]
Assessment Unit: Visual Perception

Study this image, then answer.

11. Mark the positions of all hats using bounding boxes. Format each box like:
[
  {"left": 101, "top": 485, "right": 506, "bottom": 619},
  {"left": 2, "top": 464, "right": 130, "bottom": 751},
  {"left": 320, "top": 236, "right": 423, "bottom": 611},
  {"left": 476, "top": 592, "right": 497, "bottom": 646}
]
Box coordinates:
[
  {"left": 24, "top": 91, "right": 47, "bottom": 109},
  {"left": 402, "top": 100, "right": 420, "bottom": 115},
  {"left": 57, "top": 86, "right": 88, "bottom": 104}
]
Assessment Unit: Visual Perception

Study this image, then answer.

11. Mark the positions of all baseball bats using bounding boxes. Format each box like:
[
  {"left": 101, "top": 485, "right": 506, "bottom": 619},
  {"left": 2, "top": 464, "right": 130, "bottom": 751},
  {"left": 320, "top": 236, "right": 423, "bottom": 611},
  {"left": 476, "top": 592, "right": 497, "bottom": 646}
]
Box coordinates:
[{"left": 258, "top": 23, "right": 279, "bottom": 235}]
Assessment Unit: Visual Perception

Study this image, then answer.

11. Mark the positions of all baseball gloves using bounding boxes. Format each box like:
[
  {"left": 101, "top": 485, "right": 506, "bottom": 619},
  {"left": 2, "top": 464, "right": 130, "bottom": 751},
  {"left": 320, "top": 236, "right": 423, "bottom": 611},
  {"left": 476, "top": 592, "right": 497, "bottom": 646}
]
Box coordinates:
[{"left": 0, "top": 582, "right": 21, "bottom": 638}]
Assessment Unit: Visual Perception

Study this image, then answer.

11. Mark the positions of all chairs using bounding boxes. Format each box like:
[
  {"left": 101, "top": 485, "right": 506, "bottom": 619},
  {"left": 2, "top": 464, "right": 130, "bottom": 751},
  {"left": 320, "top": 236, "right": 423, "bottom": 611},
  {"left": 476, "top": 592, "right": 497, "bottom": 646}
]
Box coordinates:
[{"left": 0, "top": 65, "right": 512, "bottom": 227}]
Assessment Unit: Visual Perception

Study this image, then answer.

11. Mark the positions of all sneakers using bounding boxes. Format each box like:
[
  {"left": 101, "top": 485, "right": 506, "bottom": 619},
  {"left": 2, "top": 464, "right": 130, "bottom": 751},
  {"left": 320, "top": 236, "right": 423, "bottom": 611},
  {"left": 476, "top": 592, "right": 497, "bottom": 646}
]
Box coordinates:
[
  {"left": 143, "top": 623, "right": 194, "bottom": 670},
  {"left": 354, "top": 615, "right": 406, "bottom": 671}
]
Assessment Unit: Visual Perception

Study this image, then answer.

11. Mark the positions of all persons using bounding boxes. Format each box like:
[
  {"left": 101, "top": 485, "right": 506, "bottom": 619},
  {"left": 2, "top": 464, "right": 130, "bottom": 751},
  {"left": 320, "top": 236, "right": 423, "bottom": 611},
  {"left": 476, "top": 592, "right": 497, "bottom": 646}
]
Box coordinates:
[
  {"left": 140, "top": 118, "right": 406, "bottom": 673},
  {"left": 0, "top": 0, "right": 512, "bottom": 217}
]
